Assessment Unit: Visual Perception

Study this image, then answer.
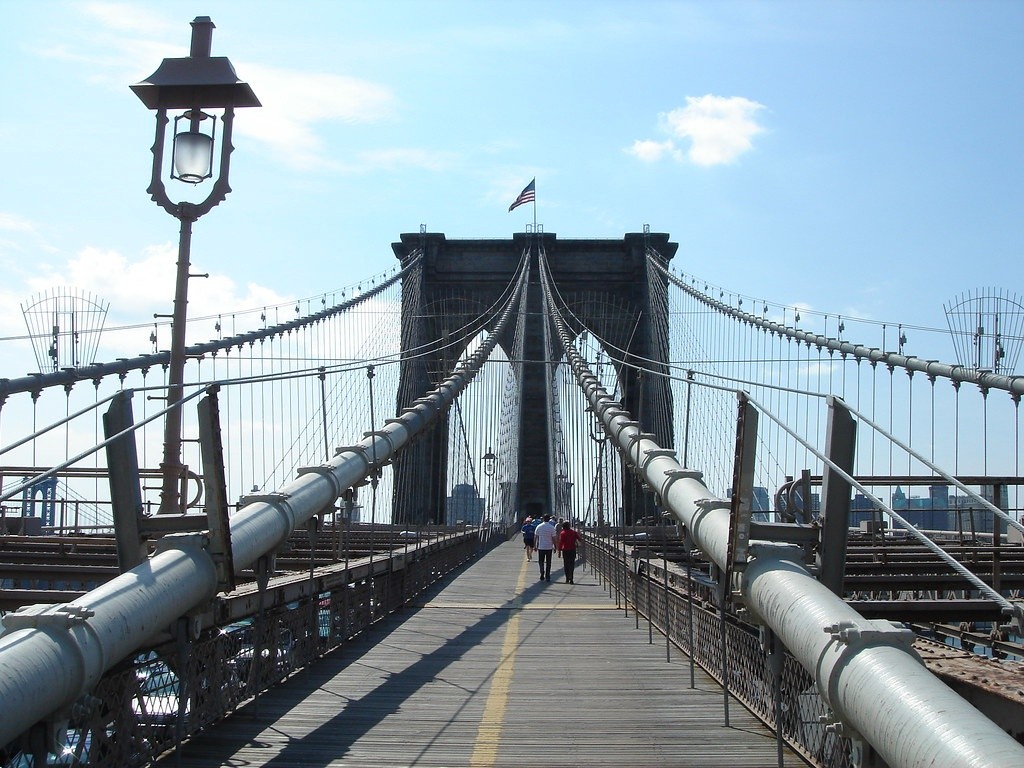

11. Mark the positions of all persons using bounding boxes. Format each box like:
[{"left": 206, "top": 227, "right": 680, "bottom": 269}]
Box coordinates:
[
  {"left": 558, "top": 521, "right": 583, "bottom": 585},
  {"left": 533, "top": 514, "right": 556, "bottom": 582},
  {"left": 521, "top": 515, "right": 581, "bottom": 562}
]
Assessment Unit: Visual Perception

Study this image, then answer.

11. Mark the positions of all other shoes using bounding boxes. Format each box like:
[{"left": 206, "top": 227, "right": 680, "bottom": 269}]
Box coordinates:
[
  {"left": 526, "top": 558, "right": 530, "bottom": 562},
  {"left": 570, "top": 579, "right": 573, "bottom": 584},
  {"left": 539, "top": 575, "right": 545, "bottom": 581},
  {"left": 566, "top": 578, "right": 569, "bottom": 583},
  {"left": 546, "top": 574, "right": 550, "bottom": 580},
  {"left": 529, "top": 555, "right": 533, "bottom": 559}
]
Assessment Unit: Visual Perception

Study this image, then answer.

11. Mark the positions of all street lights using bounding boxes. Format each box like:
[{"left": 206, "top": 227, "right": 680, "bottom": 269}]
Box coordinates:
[
  {"left": 481, "top": 447, "right": 500, "bottom": 528},
  {"left": 127, "top": 15, "right": 264, "bottom": 533}
]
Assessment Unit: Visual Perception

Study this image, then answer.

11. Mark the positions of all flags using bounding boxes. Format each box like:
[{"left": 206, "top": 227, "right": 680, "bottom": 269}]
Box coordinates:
[{"left": 509, "top": 178, "right": 535, "bottom": 212}]
